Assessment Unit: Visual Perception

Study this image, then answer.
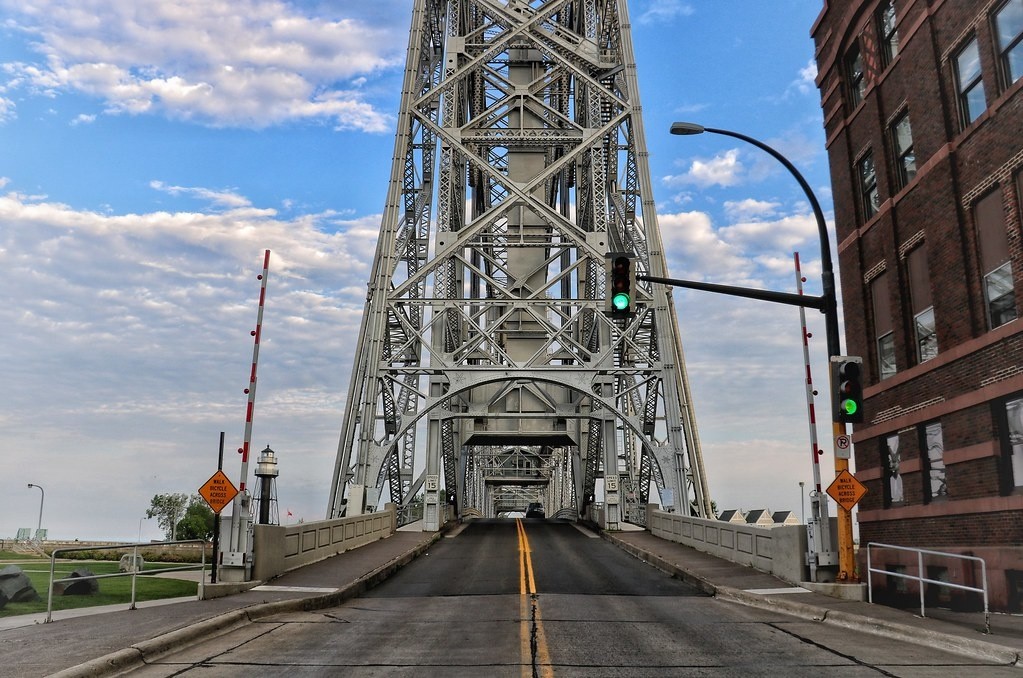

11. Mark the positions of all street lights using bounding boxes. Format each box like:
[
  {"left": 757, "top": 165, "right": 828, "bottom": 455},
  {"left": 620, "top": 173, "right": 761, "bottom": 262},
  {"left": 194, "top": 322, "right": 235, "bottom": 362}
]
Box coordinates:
[
  {"left": 138, "top": 516, "right": 147, "bottom": 543},
  {"left": 798, "top": 482, "right": 806, "bottom": 526},
  {"left": 27, "top": 483, "right": 44, "bottom": 529},
  {"left": 671, "top": 119, "right": 856, "bottom": 583}
]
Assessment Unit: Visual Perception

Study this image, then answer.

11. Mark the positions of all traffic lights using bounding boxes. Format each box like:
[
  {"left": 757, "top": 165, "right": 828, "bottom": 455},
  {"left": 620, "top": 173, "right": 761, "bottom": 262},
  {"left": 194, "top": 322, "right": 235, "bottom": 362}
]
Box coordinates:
[
  {"left": 612, "top": 255, "right": 631, "bottom": 319},
  {"left": 829, "top": 354, "right": 865, "bottom": 425}
]
